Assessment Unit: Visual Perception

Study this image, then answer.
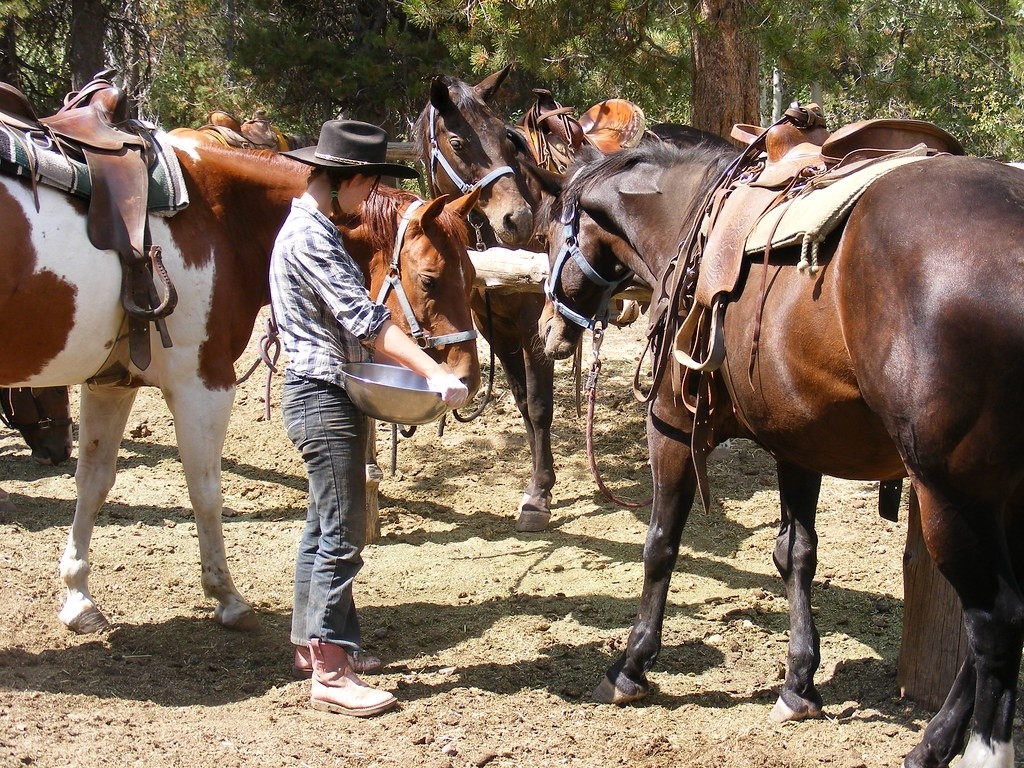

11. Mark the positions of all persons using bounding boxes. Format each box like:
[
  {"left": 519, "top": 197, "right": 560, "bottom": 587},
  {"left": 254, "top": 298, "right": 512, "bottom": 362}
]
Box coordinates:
[{"left": 268, "top": 120, "right": 469, "bottom": 718}]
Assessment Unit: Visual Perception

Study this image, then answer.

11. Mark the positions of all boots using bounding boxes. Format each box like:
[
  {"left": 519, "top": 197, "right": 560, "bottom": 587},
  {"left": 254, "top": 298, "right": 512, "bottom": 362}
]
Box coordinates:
[
  {"left": 307, "top": 638, "right": 397, "bottom": 716},
  {"left": 292, "top": 644, "right": 382, "bottom": 678}
]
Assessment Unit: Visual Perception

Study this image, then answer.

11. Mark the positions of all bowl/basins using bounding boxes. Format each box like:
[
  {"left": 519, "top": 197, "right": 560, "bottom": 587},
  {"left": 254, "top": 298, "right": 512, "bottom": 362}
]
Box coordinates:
[{"left": 336, "top": 362, "right": 467, "bottom": 425}]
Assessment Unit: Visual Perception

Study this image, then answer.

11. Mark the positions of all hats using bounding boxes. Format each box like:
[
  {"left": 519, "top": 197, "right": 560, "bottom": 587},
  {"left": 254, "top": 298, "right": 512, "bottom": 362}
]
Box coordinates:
[{"left": 278, "top": 120, "right": 422, "bottom": 179}]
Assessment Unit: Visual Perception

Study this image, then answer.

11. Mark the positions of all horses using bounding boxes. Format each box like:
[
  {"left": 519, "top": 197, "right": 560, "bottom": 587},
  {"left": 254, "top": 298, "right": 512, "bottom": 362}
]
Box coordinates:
[{"left": 0, "top": 63, "right": 1024, "bottom": 768}]
